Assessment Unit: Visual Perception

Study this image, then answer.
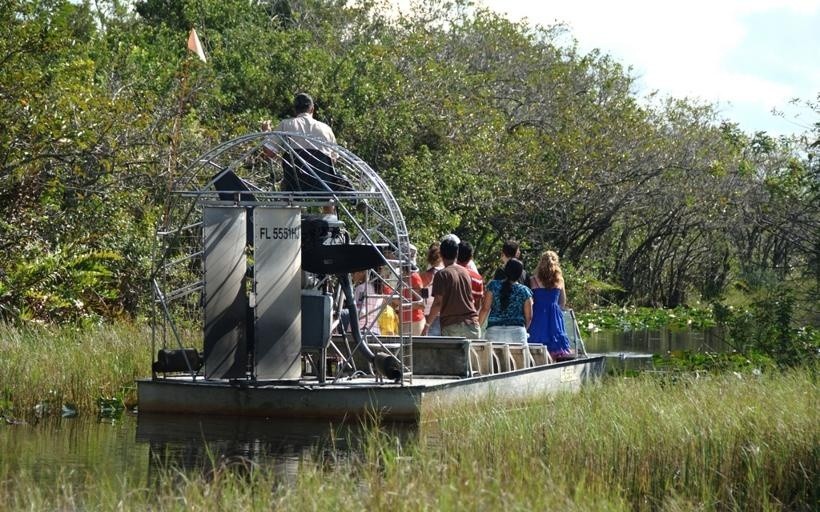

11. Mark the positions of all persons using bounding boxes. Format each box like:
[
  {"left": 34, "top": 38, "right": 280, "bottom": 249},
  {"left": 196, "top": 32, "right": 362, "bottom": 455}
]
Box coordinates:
[
  {"left": 260, "top": 94, "right": 348, "bottom": 226},
  {"left": 333, "top": 231, "right": 575, "bottom": 363}
]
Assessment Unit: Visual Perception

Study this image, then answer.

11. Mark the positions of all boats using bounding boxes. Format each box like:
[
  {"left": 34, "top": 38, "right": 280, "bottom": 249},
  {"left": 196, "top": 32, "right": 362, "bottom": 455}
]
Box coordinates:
[
  {"left": 132, "top": 122, "right": 607, "bottom": 426},
  {"left": 126, "top": 416, "right": 413, "bottom": 496}
]
